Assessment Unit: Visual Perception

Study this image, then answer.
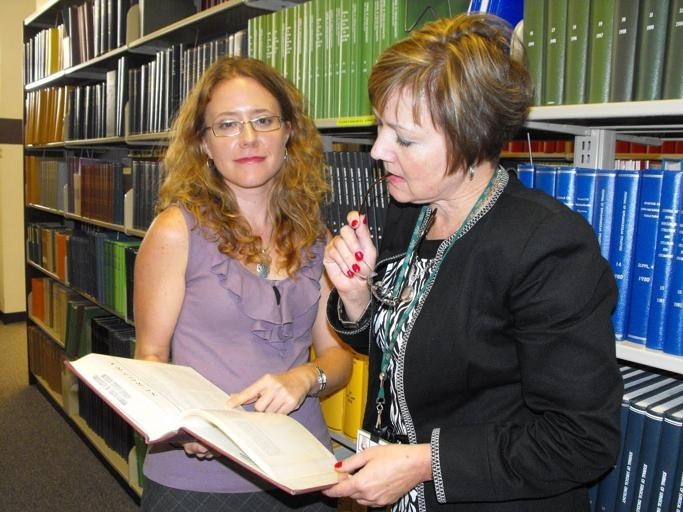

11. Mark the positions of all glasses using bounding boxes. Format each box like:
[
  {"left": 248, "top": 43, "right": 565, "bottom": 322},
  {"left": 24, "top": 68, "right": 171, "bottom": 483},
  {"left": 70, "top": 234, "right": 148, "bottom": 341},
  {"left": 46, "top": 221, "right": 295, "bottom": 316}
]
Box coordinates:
[
  {"left": 204, "top": 116, "right": 288, "bottom": 138},
  {"left": 349, "top": 172, "right": 438, "bottom": 303}
]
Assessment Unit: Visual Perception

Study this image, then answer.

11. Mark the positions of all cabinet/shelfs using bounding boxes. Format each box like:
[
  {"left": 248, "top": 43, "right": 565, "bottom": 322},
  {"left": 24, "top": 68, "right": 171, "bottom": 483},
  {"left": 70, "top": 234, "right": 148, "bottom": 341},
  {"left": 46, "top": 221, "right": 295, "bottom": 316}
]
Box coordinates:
[{"left": 24, "top": 0, "right": 681, "bottom": 512}]
